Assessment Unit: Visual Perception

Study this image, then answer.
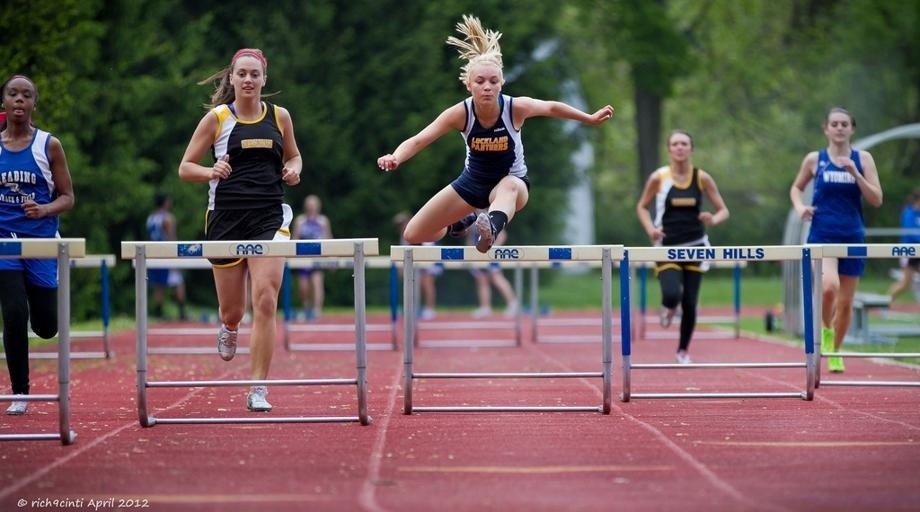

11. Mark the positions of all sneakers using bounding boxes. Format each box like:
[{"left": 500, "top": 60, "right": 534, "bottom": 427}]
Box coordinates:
[
  {"left": 660, "top": 305, "right": 675, "bottom": 328},
  {"left": 449, "top": 211, "right": 477, "bottom": 239},
  {"left": 827, "top": 355, "right": 845, "bottom": 372},
  {"left": 5, "top": 396, "right": 28, "bottom": 415},
  {"left": 216, "top": 322, "right": 239, "bottom": 362},
  {"left": 474, "top": 211, "right": 496, "bottom": 253},
  {"left": 821, "top": 325, "right": 835, "bottom": 356},
  {"left": 675, "top": 348, "right": 691, "bottom": 364},
  {"left": 245, "top": 384, "right": 272, "bottom": 412}
]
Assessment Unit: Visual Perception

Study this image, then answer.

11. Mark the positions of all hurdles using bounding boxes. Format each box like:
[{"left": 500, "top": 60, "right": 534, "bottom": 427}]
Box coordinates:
[
  {"left": 390, "top": 244, "right": 626, "bottom": 417},
  {"left": 0, "top": 238, "right": 87, "bottom": 446},
  {"left": 1, "top": 254, "right": 116, "bottom": 359},
  {"left": 804, "top": 242, "right": 920, "bottom": 386},
  {"left": 620, "top": 244, "right": 822, "bottom": 401},
  {"left": 130, "top": 255, "right": 399, "bottom": 385},
  {"left": 632, "top": 260, "right": 919, "bottom": 370},
  {"left": 392, "top": 259, "right": 621, "bottom": 379},
  {"left": 121, "top": 238, "right": 380, "bottom": 427}
]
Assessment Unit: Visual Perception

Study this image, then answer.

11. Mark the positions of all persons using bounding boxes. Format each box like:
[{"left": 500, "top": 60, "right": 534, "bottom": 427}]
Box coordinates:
[
  {"left": 1, "top": 76, "right": 77, "bottom": 416},
  {"left": 889, "top": 188, "right": 920, "bottom": 301},
  {"left": 636, "top": 130, "right": 732, "bottom": 366},
  {"left": 147, "top": 193, "right": 526, "bottom": 322},
  {"left": 375, "top": 14, "right": 614, "bottom": 251},
  {"left": 180, "top": 49, "right": 304, "bottom": 413},
  {"left": 789, "top": 105, "right": 884, "bottom": 376}
]
